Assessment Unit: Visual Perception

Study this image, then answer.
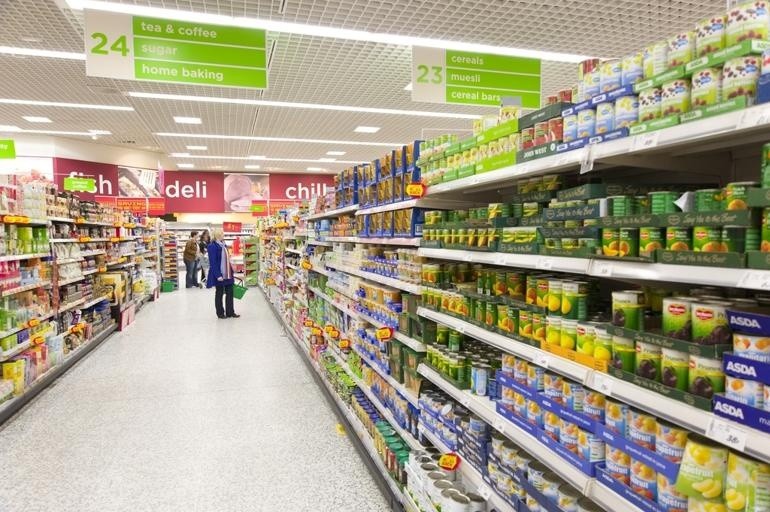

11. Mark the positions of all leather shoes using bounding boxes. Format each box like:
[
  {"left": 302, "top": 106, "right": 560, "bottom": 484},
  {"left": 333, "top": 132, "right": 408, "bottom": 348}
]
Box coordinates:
[
  {"left": 218, "top": 315, "right": 227, "bottom": 319},
  {"left": 226, "top": 313, "right": 240, "bottom": 318}
]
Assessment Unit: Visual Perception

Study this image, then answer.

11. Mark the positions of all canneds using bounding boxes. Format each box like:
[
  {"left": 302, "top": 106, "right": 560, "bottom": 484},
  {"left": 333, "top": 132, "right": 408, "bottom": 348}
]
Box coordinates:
[
  {"left": 414, "top": 5, "right": 770, "bottom": 189},
  {"left": 355, "top": 249, "right": 770, "bottom": 431},
  {"left": 355, "top": 421, "right": 770, "bottom": 511},
  {"left": 416, "top": 141, "right": 770, "bottom": 270}
]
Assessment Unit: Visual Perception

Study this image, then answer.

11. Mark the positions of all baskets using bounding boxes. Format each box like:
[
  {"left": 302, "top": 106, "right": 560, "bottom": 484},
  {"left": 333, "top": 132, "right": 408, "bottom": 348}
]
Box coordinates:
[
  {"left": 161, "top": 282, "right": 176, "bottom": 292},
  {"left": 222, "top": 276, "right": 248, "bottom": 299}
]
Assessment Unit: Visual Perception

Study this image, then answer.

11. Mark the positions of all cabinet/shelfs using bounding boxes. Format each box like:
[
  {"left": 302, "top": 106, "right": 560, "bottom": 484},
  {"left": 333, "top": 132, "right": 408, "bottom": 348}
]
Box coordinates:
[
  {"left": 1, "top": 179, "right": 161, "bottom": 421},
  {"left": 161, "top": 218, "right": 258, "bottom": 292}
]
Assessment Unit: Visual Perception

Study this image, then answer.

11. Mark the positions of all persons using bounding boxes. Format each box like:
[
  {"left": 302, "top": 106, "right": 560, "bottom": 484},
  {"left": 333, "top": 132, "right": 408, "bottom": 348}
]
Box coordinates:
[
  {"left": 204, "top": 230, "right": 210, "bottom": 244},
  {"left": 198, "top": 236, "right": 209, "bottom": 282},
  {"left": 207, "top": 229, "right": 240, "bottom": 318},
  {"left": 183, "top": 231, "right": 199, "bottom": 288}
]
